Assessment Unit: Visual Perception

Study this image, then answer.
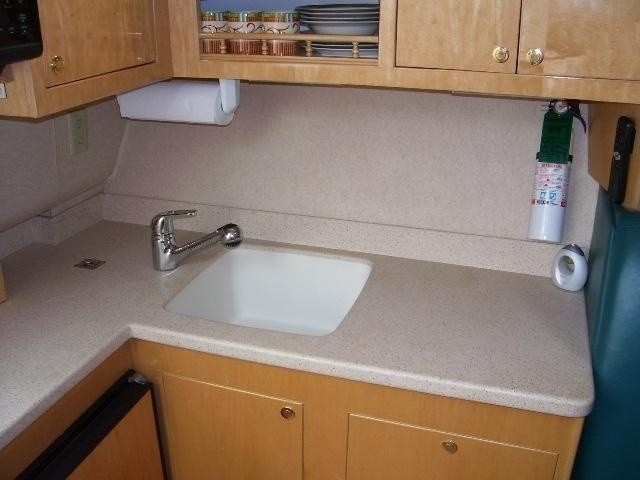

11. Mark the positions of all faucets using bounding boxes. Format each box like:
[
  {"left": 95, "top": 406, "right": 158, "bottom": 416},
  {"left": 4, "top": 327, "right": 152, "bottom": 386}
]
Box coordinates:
[{"left": 150, "top": 209, "right": 245, "bottom": 272}]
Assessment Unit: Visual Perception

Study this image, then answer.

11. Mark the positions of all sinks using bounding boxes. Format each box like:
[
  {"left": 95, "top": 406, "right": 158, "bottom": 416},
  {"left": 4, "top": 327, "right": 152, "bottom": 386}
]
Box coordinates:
[{"left": 163, "top": 242, "right": 375, "bottom": 337}]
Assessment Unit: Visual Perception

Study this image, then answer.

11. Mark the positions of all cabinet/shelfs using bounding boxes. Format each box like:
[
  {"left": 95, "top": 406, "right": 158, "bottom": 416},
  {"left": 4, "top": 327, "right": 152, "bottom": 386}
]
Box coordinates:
[
  {"left": 387, "top": 1, "right": 640, "bottom": 108},
  {"left": 1, "top": 345, "right": 163, "bottom": 479},
  {"left": 161, "top": 345, "right": 585, "bottom": 480},
  {"left": 170, "top": 0, "right": 387, "bottom": 87},
  {"left": 0, "top": 2, "right": 169, "bottom": 117}
]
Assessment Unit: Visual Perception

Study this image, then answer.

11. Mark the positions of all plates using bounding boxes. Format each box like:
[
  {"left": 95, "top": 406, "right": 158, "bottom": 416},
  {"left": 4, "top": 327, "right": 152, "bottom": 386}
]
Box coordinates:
[{"left": 294, "top": 3, "right": 378, "bottom": 58}]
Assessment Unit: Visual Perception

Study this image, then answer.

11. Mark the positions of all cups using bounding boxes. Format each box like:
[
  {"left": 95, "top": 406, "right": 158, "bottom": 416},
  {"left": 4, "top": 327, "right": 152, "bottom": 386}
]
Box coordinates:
[{"left": 197, "top": 10, "right": 301, "bottom": 57}]
